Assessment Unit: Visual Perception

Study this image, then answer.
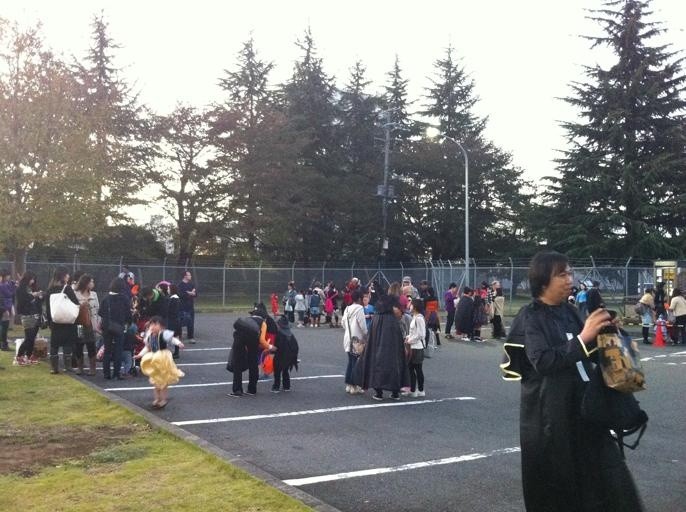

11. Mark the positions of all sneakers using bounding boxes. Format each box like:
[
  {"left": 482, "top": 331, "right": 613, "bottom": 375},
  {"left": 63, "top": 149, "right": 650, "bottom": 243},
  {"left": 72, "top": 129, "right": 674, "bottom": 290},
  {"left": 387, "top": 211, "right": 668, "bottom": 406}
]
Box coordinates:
[
  {"left": 346, "top": 385, "right": 425, "bottom": 401},
  {"left": 14, "top": 354, "right": 38, "bottom": 366},
  {"left": 444, "top": 335, "right": 487, "bottom": 343}
]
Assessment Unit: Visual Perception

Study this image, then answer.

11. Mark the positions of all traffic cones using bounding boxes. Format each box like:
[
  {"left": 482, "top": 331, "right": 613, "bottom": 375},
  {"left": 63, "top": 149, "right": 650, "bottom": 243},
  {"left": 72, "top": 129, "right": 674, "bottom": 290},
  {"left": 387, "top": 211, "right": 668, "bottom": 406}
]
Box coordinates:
[{"left": 652, "top": 321, "right": 666, "bottom": 347}]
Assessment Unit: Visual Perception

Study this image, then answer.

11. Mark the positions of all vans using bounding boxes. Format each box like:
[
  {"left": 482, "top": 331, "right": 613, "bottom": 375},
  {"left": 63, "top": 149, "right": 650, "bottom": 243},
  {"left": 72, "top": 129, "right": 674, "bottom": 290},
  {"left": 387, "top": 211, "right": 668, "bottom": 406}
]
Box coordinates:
[{"left": 638, "top": 283, "right": 654, "bottom": 293}]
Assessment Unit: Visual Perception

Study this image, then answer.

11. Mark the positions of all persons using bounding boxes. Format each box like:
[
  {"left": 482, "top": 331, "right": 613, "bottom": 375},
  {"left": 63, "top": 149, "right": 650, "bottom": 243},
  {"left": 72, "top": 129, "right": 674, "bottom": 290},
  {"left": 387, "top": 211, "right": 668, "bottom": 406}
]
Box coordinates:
[
  {"left": 226, "top": 304, "right": 299, "bottom": 397},
  {"left": 635, "top": 287, "right": 659, "bottom": 344},
  {"left": 271, "top": 275, "right": 442, "bottom": 345},
  {"left": 500, "top": 251, "right": 649, "bottom": 512},
  {"left": 650, "top": 282, "right": 667, "bottom": 328},
  {"left": 663, "top": 288, "right": 686, "bottom": 345},
  {"left": 444, "top": 282, "right": 506, "bottom": 344},
  {"left": 132, "top": 316, "right": 186, "bottom": 411},
  {"left": 1, "top": 265, "right": 197, "bottom": 380},
  {"left": 575, "top": 283, "right": 587, "bottom": 315},
  {"left": 340, "top": 286, "right": 427, "bottom": 401}
]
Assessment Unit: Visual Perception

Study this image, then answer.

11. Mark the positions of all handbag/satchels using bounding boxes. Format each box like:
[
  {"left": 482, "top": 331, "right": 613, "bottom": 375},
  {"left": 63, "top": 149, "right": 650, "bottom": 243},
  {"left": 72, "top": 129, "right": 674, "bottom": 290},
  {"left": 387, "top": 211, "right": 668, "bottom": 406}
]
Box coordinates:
[
  {"left": 635, "top": 303, "right": 646, "bottom": 315},
  {"left": 350, "top": 336, "right": 365, "bottom": 357},
  {"left": 75, "top": 302, "right": 90, "bottom": 327},
  {"left": 581, "top": 365, "right": 648, "bottom": 450},
  {"left": 233, "top": 316, "right": 260, "bottom": 338},
  {"left": 596, "top": 332, "right": 646, "bottom": 394},
  {"left": 49, "top": 293, "right": 81, "bottom": 324},
  {"left": 108, "top": 321, "right": 124, "bottom": 337}
]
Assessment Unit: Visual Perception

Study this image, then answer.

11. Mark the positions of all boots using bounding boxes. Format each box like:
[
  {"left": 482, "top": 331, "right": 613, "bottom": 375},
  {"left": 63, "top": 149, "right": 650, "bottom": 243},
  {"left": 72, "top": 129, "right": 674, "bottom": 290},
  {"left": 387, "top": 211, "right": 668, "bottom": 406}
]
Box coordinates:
[{"left": 49, "top": 353, "right": 126, "bottom": 379}]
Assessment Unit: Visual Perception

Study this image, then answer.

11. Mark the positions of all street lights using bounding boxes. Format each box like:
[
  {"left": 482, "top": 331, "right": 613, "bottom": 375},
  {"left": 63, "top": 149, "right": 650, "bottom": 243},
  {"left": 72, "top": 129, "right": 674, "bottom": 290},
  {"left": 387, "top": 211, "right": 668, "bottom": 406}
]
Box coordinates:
[{"left": 425, "top": 126, "right": 470, "bottom": 288}]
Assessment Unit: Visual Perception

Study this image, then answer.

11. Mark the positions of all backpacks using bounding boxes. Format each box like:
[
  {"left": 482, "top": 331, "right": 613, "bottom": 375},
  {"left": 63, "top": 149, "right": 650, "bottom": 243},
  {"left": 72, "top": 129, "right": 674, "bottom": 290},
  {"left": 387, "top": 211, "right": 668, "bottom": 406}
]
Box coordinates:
[{"left": 415, "top": 316, "right": 435, "bottom": 358}]
